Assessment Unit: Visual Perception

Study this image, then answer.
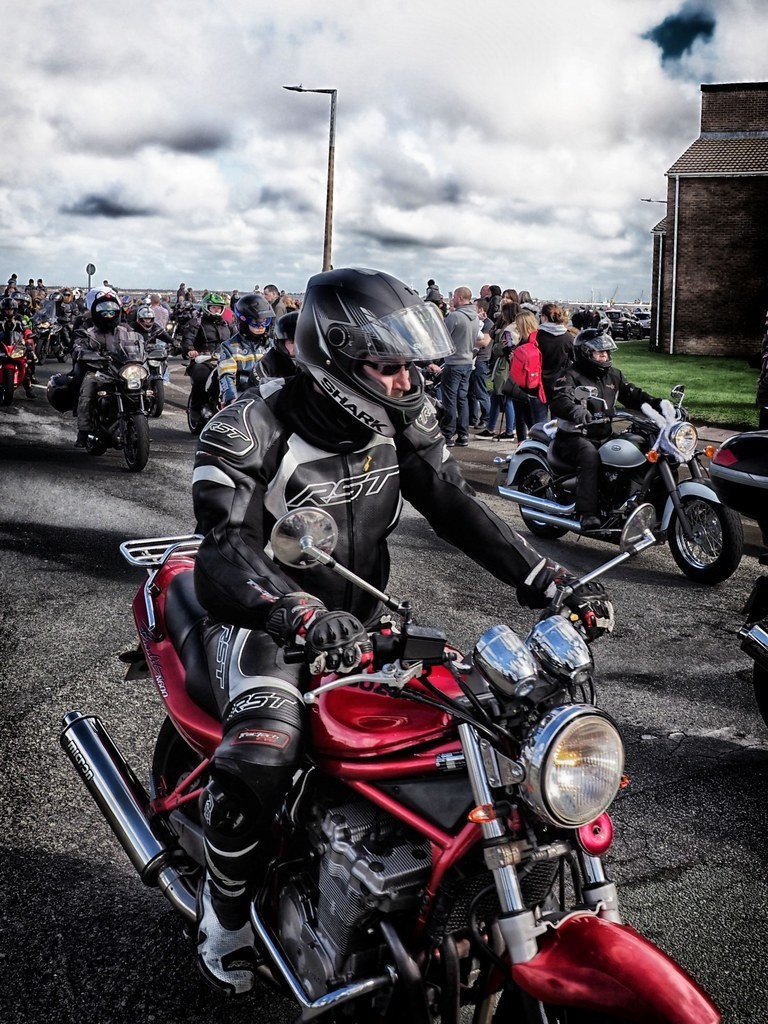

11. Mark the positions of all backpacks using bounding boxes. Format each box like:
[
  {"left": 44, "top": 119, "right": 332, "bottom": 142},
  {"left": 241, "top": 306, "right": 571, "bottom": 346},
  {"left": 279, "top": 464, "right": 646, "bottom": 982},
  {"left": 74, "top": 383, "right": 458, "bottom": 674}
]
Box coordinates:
[{"left": 507, "top": 331, "right": 546, "bottom": 404}]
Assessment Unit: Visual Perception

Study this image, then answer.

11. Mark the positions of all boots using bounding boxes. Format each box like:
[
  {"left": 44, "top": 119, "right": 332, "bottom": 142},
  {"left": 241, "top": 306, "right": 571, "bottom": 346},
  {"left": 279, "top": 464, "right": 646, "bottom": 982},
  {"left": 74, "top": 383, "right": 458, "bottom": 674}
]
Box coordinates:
[{"left": 180, "top": 784, "right": 276, "bottom": 1013}]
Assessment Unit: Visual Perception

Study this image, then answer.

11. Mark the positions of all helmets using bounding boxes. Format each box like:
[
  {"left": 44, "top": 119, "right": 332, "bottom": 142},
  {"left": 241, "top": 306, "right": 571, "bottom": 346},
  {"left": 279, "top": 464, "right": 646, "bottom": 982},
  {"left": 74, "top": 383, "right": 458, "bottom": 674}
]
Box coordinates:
[
  {"left": 202, "top": 294, "right": 226, "bottom": 319},
  {"left": 90, "top": 295, "right": 120, "bottom": 334},
  {"left": 293, "top": 268, "right": 433, "bottom": 438},
  {"left": 138, "top": 306, "right": 155, "bottom": 331},
  {"left": 0, "top": 289, "right": 70, "bottom": 309},
  {"left": 572, "top": 329, "right": 613, "bottom": 377},
  {"left": 234, "top": 295, "right": 273, "bottom": 341},
  {"left": 274, "top": 310, "right": 299, "bottom": 366}
]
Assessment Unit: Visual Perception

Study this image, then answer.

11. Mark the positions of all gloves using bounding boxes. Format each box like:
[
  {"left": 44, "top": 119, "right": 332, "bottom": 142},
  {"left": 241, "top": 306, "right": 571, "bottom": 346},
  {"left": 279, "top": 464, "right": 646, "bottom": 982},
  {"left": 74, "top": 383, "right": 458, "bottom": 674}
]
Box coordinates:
[
  {"left": 251, "top": 589, "right": 385, "bottom": 691},
  {"left": 572, "top": 406, "right": 592, "bottom": 429},
  {"left": 521, "top": 559, "right": 626, "bottom": 646}
]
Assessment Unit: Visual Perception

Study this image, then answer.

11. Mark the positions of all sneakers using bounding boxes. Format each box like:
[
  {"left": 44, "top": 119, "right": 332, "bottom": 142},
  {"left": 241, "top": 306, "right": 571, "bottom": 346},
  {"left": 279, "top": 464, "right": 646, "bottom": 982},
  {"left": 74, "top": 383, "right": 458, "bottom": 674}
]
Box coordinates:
[{"left": 475, "top": 430, "right": 514, "bottom": 442}]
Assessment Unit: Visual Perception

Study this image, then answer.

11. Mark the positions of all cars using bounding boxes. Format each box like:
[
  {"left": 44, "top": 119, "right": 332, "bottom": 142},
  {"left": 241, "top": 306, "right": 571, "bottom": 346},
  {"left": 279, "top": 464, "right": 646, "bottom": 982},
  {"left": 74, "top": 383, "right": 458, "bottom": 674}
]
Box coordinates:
[{"left": 580, "top": 308, "right": 652, "bottom": 340}]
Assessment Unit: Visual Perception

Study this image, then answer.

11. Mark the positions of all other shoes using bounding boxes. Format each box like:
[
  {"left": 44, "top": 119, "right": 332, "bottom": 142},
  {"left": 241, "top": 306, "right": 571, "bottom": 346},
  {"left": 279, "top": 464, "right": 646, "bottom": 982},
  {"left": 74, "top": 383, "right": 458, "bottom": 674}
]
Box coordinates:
[
  {"left": 30, "top": 374, "right": 40, "bottom": 385},
  {"left": 579, "top": 516, "right": 601, "bottom": 530},
  {"left": 445, "top": 438, "right": 468, "bottom": 446},
  {"left": 190, "top": 411, "right": 202, "bottom": 423},
  {"left": 26, "top": 390, "right": 38, "bottom": 398}
]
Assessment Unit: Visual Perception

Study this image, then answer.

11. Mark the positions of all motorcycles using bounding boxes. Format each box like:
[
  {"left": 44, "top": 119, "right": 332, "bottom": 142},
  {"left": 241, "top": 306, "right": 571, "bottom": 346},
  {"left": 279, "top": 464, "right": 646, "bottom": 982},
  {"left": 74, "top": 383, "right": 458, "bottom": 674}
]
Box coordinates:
[
  {"left": 419, "top": 366, "right": 450, "bottom": 442},
  {"left": 46, "top": 329, "right": 167, "bottom": 472},
  {"left": 0, "top": 328, "right": 37, "bottom": 408},
  {"left": 30, "top": 299, "right": 82, "bottom": 365},
  {"left": 182, "top": 350, "right": 225, "bottom": 436},
  {"left": 122, "top": 309, "right": 195, "bottom": 417},
  {"left": 620, "top": 312, "right": 644, "bottom": 341},
  {"left": 708, "top": 428, "right": 768, "bottom": 730},
  {"left": 62, "top": 502, "right": 721, "bottom": 1024},
  {"left": 491, "top": 384, "right": 743, "bottom": 588}
]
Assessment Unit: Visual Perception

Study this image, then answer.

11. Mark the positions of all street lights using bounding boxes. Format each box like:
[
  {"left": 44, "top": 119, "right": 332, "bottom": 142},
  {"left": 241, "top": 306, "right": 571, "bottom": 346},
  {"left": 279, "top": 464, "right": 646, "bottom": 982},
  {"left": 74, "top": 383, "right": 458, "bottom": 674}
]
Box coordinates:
[{"left": 283, "top": 86, "right": 337, "bottom": 269}]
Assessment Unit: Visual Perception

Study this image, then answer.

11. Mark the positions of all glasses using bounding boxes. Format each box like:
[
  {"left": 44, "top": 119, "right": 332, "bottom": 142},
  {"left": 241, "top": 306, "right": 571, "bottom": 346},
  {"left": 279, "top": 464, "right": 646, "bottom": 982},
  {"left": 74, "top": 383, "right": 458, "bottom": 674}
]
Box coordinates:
[
  {"left": 359, "top": 360, "right": 433, "bottom": 376},
  {"left": 101, "top": 310, "right": 115, "bottom": 316},
  {"left": 538, "top": 314, "right": 542, "bottom": 319}
]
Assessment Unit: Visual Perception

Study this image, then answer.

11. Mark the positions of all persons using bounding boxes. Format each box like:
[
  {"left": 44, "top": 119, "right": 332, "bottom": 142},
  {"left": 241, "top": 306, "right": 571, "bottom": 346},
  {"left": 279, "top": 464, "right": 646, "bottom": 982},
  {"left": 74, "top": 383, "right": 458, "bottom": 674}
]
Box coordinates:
[
  {"left": 413, "top": 279, "right": 650, "bottom": 447},
  {"left": 192, "top": 267, "right": 615, "bottom": 997},
  {"left": 550, "top": 328, "right": 688, "bottom": 531},
  {"left": 0, "top": 273, "right": 303, "bottom": 449}
]
